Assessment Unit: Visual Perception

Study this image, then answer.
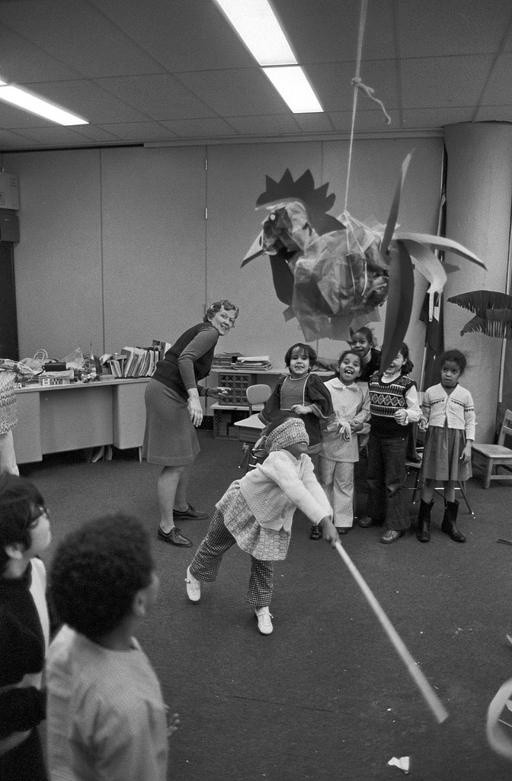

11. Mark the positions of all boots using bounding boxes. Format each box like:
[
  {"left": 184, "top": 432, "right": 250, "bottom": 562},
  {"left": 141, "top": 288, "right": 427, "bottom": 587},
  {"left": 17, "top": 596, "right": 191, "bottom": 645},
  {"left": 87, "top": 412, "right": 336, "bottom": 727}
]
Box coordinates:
[
  {"left": 416, "top": 498, "right": 435, "bottom": 542},
  {"left": 441, "top": 499, "right": 466, "bottom": 542}
]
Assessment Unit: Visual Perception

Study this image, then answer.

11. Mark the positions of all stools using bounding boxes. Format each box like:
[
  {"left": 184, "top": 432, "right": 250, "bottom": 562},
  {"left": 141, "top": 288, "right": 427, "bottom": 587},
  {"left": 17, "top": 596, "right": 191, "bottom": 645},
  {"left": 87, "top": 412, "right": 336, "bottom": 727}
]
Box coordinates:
[{"left": 404, "top": 452, "right": 475, "bottom": 516}]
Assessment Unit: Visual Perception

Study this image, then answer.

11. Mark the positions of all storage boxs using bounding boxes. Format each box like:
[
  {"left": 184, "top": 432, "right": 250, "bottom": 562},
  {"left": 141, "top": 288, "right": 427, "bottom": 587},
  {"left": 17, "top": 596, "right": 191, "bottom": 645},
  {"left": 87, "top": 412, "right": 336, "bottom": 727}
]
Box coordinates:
[{"left": 217, "top": 372, "right": 257, "bottom": 406}]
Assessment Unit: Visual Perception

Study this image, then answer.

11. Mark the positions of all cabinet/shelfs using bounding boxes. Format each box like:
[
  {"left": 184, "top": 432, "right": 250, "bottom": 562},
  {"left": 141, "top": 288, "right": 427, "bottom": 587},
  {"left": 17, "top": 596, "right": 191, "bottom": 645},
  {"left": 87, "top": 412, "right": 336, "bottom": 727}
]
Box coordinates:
[{"left": 208, "top": 368, "right": 336, "bottom": 441}]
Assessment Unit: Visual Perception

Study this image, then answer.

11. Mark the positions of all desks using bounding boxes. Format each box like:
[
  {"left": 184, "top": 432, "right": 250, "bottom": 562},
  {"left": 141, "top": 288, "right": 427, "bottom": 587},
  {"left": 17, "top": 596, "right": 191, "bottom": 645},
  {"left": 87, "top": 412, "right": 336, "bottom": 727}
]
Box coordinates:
[
  {"left": 234, "top": 412, "right": 266, "bottom": 465},
  {"left": 11, "top": 374, "right": 151, "bottom": 465}
]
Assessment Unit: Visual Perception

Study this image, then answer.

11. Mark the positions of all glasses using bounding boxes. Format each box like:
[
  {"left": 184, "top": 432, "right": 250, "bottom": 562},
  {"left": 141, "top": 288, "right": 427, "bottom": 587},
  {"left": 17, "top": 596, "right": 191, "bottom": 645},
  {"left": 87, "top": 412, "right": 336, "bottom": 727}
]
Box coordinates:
[{"left": 31, "top": 504, "right": 49, "bottom": 523}]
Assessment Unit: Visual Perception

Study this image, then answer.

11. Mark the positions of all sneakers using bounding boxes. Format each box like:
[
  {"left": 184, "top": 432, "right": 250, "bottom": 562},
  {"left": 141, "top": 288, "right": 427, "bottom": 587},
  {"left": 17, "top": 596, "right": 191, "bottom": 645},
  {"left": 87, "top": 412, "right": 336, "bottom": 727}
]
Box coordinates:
[
  {"left": 311, "top": 526, "right": 322, "bottom": 540},
  {"left": 337, "top": 527, "right": 347, "bottom": 534},
  {"left": 359, "top": 516, "right": 374, "bottom": 528},
  {"left": 380, "top": 530, "right": 403, "bottom": 542}
]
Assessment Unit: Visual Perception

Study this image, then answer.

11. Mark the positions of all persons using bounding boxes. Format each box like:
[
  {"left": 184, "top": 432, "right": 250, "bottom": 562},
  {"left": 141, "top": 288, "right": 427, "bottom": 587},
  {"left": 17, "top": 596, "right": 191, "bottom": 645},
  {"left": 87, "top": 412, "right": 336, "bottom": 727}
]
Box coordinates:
[
  {"left": 418, "top": 350, "right": 476, "bottom": 543},
  {"left": 140, "top": 299, "right": 240, "bottom": 547},
  {"left": 307, "top": 351, "right": 371, "bottom": 540},
  {"left": 258, "top": 342, "right": 333, "bottom": 480},
  {"left": 0, "top": 473, "right": 54, "bottom": 781},
  {"left": 185, "top": 414, "right": 342, "bottom": 634},
  {"left": 349, "top": 326, "right": 422, "bottom": 463},
  {"left": 350, "top": 341, "right": 423, "bottom": 543},
  {"left": 47, "top": 511, "right": 168, "bottom": 781}
]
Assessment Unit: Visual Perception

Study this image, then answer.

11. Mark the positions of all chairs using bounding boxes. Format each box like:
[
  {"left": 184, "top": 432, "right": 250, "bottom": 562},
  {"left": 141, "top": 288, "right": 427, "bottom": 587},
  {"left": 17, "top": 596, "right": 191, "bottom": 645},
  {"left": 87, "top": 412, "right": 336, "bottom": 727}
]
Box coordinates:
[
  {"left": 471, "top": 408, "right": 512, "bottom": 489},
  {"left": 237, "top": 383, "right": 272, "bottom": 469}
]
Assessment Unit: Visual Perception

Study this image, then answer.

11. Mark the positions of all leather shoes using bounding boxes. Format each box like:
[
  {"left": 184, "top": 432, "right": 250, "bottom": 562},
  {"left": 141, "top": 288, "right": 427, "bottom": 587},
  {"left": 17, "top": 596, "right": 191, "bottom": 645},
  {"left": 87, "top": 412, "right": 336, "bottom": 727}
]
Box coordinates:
[
  {"left": 174, "top": 506, "right": 208, "bottom": 521},
  {"left": 255, "top": 606, "right": 274, "bottom": 635},
  {"left": 184, "top": 567, "right": 201, "bottom": 602},
  {"left": 158, "top": 526, "right": 192, "bottom": 547}
]
